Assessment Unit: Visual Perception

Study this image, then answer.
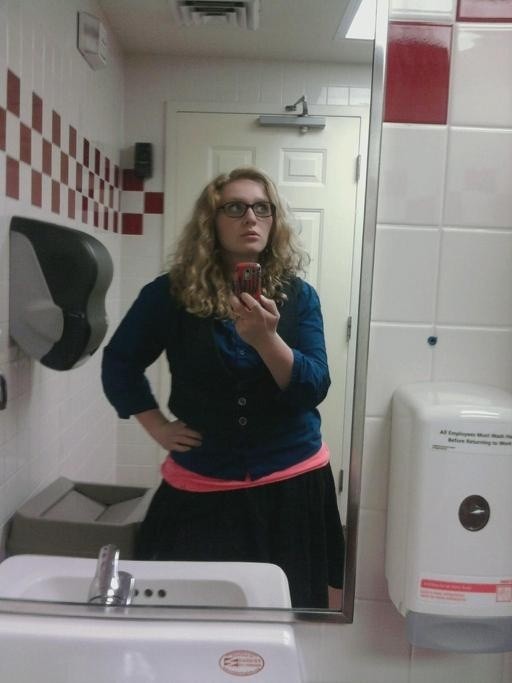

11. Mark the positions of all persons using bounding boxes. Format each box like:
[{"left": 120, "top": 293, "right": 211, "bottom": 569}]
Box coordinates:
[{"left": 98, "top": 161, "right": 345, "bottom": 615}]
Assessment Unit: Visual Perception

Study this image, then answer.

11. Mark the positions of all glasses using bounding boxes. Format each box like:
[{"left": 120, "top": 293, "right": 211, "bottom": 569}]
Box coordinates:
[{"left": 212, "top": 199, "right": 278, "bottom": 217}]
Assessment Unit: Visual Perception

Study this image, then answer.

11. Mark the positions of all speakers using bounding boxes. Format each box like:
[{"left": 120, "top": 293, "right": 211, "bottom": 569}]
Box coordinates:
[{"left": 134, "top": 143, "right": 153, "bottom": 179}]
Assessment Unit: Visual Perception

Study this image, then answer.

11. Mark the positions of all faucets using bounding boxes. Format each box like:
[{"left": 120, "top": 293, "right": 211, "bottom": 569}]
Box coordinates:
[{"left": 87, "top": 545, "right": 135, "bottom": 608}]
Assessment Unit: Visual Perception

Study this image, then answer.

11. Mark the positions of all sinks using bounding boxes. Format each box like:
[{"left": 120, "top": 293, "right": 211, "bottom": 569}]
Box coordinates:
[
  {"left": 0, "top": 554, "right": 293, "bottom": 608},
  {"left": 0, "top": 613, "right": 303, "bottom": 683}
]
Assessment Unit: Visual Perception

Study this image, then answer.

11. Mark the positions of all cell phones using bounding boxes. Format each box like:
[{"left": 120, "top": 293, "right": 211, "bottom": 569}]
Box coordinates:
[{"left": 234, "top": 263, "right": 261, "bottom": 305}]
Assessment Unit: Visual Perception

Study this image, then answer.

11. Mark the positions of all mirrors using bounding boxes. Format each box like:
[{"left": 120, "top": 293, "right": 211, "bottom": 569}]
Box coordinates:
[{"left": 0, "top": 1, "right": 390, "bottom": 625}]
